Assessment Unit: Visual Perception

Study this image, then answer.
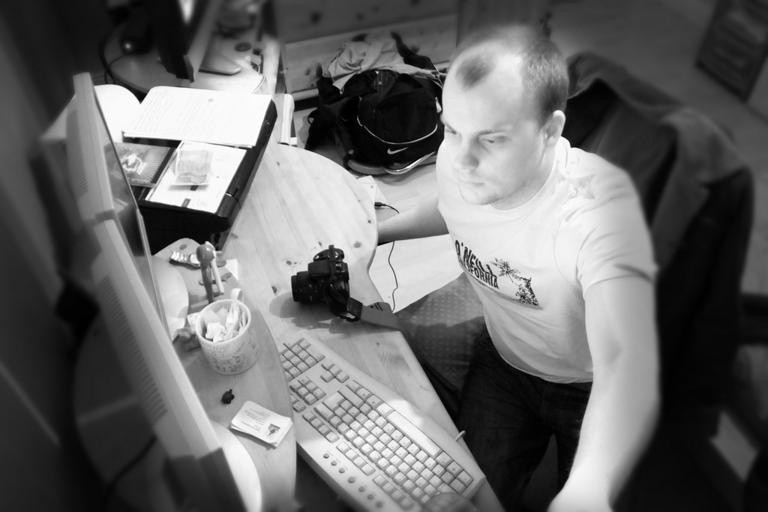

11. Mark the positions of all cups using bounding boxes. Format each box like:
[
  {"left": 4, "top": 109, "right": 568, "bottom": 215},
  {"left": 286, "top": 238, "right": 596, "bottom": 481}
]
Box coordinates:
[
  {"left": 422, "top": 491, "right": 478, "bottom": 512},
  {"left": 195, "top": 288, "right": 258, "bottom": 375}
]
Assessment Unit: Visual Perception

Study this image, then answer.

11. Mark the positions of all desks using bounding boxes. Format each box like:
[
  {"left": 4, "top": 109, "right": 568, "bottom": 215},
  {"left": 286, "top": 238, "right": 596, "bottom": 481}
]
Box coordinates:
[{"left": 63, "top": 28, "right": 509, "bottom": 510}]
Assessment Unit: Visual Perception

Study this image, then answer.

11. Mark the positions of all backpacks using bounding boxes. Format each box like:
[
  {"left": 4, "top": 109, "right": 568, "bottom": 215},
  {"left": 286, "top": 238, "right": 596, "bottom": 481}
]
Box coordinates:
[{"left": 304, "top": 30, "right": 448, "bottom": 176}]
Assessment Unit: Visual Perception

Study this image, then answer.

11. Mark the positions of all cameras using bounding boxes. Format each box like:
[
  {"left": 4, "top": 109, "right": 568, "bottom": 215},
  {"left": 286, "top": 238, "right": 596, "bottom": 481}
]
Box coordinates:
[{"left": 292, "top": 245, "right": 362, "bottom": 322}]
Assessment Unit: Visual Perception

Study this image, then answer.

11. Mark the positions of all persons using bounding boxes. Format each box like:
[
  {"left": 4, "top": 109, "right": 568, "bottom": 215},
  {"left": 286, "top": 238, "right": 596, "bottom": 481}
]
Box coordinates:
[{"left": 377, "top": 22, "right": 664, "bottom": 512}]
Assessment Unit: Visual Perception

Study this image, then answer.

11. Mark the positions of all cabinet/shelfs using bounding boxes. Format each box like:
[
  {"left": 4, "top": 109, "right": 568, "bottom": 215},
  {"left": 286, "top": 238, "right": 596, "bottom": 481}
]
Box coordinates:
[
  {"left": 264, "top": 0, "right": 460, "bottom": 103},
  {"left": 697, "top": 0, "right": 768, "bottom": 103}
]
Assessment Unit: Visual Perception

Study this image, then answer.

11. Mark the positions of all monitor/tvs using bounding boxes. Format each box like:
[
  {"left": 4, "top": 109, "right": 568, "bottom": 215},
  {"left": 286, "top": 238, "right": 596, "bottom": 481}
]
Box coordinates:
[
  {"left": 90, "top": 219, "right": 263, "bottom": 512},
  {"left": 151, "top": 0, "right": 240, "bottom": 83},
  {"left": 66, "top": 71, "right": 189, "bottom": 346}
]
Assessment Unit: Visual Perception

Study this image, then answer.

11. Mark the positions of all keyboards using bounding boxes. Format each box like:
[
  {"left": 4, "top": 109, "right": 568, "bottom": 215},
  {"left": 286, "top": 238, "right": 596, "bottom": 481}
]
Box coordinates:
[{"left": 276, "top": 329, "right": 486, "bottom": 512}]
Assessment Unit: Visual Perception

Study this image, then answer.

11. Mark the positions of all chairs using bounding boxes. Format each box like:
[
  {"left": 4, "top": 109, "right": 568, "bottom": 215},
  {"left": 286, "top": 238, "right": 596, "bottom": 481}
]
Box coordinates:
[{"left": 394, "top": 78, "right": 755, "bottom": 511}]
